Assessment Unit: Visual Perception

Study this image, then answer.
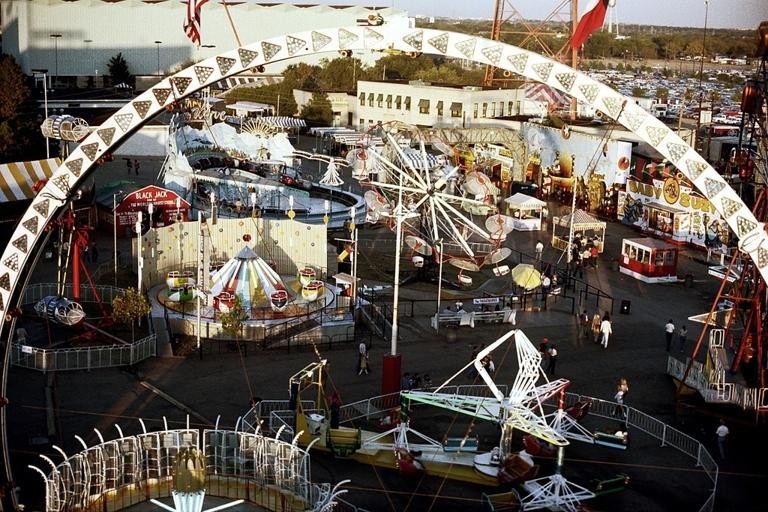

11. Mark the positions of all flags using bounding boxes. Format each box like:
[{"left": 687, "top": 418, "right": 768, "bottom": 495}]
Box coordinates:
[
  {"left": 569, "top": 0, "right": 608, "bottom": 52},
  {"left": 182, "top": 0, "right": 210, "bottom": 51}
]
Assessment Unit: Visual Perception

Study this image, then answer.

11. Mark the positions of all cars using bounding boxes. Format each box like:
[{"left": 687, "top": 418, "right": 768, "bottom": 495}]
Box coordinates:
[{"left": 585, "top": 69, "right": 766, "bottom": 137}]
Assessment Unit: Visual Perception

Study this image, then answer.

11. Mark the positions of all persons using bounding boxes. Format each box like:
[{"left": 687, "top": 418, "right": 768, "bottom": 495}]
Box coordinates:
[
  {"left": 677, "top": 325, "right": 688, "bottom": 353},
  {"left": 127, "top": 159, "right": 133, "bottom": 175},
  {"left": 470, "top": 343, "right": 494, "bottom": 382},
  {"left": 134, "top": 159, "right": 139, "bottom": 176},
  {"left": 311, "top": 139, "right": 339, "bottom": 162},
  {"left": 455, "top": 299, "right": 463, "bottom": 312},
  {"left": 514, "top": 207, "right": 548, "bottom": 230},
  {"left": 442, "top": 306, "right": 453, "bottom": 328},
  {"left": 200, "top": 192, "right": 242, "bottom": 218},
  {"left": 484, "top": 303, "right": 511, "bottom": 315},
  {"left": 457, "top": 307, "right": 467, "bottom": 325},
  {"left": 572, "top": 232, "right": 601, "bottom": 270},
  {"left": 401, "top": 372, "right": 433, "bottom": 410},
  {"left": 562, "top": 234, "right": 569, "bottom": 242},
  {"left": 16, "top": 324, "right": 29, "bottom": 363},
  {"left": 537, "top": 337, "right": 558, "bottom": 376},
  {"left": 627, "top": 245, "right": 635, "bottom": 258},
  {"left": 396, "top": 453, "right": 425, "bottom": 495},
  {"left": 541, "top": 271, "right": 550, "bottom": 310},
  {"left": 323, "top": 337, "right": 370, "bottom": 393},
  {"left": 663, "top": 319, "right": 675, "bottom": 352},
  {"left": 609, "top": 256, "right": 619, "bottom": 264},
  {"left": 578, "top": 310, "right": 612, "bottom": 348},
  {"left": 709, "top": 220, "right": 724, "bottom": 248},
  {"left": 536, "top": 239, "right": 543, "bottom": 262},
  {"left": 168, "top": 103, "right": 188, "bottom": 113},
  {"left": 307, "top": 207, "right": 311, "bottom": 216},
  {"left": 715, "top": 418, "right": 730, "bottom": 461},
  {"left": 613, "top": 378, "right": 628, "bottom": 418}
]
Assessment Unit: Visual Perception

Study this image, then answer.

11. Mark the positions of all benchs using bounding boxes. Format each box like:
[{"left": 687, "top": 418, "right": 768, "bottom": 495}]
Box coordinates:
[
  {"left": 473, "top": 310, "right": 516, "bottom": 327},
  {"left": 431, "top": 311, "right": 473, "bottom": 330}
]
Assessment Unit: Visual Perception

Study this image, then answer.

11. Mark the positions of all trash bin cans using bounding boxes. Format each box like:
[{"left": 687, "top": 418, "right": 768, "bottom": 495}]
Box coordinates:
[{"left": 621, "top": 300, "right": 631, "bottom": 314}]
